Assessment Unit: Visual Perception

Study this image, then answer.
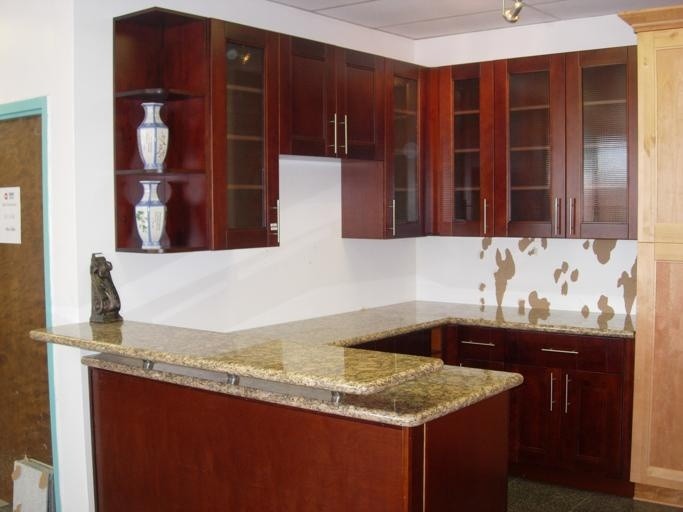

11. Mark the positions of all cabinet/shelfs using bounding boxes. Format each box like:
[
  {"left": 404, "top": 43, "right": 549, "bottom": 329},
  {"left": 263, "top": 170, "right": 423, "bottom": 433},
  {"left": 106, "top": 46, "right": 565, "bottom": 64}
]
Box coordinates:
[
  {"left": 492, "top": 44, "right": 637, "bottom": 241},
  {"left": 618, "top": 3, "right": 683, "bottom": 511},
  {"left": 383, "top": 57, "right": 427, "bottom": 240},
  {"left": 507, "top": 331, "right": 626, "bottom": 479},
  {"left": 455, "top": 326, "right": 505, "bottom": 374},
  {"left": 113, "top": 8, "right": 211, "bottom": 255},
  {"left": 280, "top": 33, "right": 386, "bottom": 164},
  {"left": 210, "top": 17, "right": 282, "bottom": 253},
  {"left": 435, "top": 61, "right": 494, "bottom": 239}
]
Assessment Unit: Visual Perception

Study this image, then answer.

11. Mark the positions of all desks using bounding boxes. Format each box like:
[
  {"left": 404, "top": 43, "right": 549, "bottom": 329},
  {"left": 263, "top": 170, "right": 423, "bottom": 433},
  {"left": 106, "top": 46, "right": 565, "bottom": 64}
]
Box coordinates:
[
  {"left": 23, "top": 320, "right": 523, "bottom": 510},
  {"left": 226, "top": 299, "right": 640, "bottom": 348}
]
